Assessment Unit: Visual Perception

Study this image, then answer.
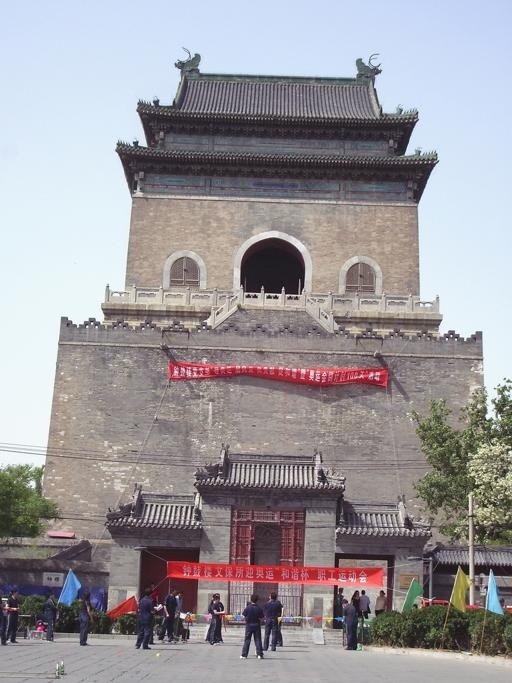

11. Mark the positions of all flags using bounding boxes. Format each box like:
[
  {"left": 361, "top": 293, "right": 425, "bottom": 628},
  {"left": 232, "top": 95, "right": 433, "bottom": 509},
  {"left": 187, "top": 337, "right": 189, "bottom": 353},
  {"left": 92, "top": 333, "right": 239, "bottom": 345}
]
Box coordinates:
[
  {"left": 450, "top": 565, "right": 473, "bottom": 613},
  {"left": 485, "top": 568, "right": 505, "bottom": 616},
  {"left": 58, "top": 569, "right": 83, "bottom": 607},
  {"left": 106, "top": 595, "right": 139, "bottom": 621},
  {"left": 401, "top": 577, "right": 425, "bottom": 616}
]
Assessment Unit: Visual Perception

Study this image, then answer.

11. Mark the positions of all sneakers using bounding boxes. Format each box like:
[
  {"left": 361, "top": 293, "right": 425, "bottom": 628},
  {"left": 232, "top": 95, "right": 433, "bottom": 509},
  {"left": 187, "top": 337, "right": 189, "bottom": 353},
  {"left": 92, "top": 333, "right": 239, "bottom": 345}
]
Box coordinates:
[
  {"left": 257, "top": 655, "right": 264, "bottom": 659},
  {"left": 344, "top": 646, "right": 357, "bottom": 650},
  {"left": 1, "top": 640, "right": 20, "bottom": 646},
  {"left": 239, "top": 656, "right": 246, "bottom": 659},
  {"left": 157, "top": 638, "right": 188, "bottom": 643},
  {"left": 79, "top": 642, "right": 88, "bottom": 646},
  {"left": 205, "top": 639, "right": 224, "bottom": 646},
  {"left": 45, "top": 640, "right": 56, "bottom": 642},
  {"left": 134, "top": 645, "right": 152, "bottom": 649}
]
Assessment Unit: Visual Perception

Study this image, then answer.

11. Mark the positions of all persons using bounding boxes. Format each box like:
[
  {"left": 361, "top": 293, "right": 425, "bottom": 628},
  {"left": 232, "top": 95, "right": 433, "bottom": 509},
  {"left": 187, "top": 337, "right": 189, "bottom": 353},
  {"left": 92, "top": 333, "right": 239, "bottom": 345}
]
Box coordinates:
[
  {"left": 0, "top": 594, "right": 10, "bottom": 645},
  {"left": 261, "top": 592, "right": 282, "bottom": 652},
  {"left": 270, "top": 598, "right": 284, "bottom": 646},
  {"left": 333, "top": 584, "right": 387, "bottom": 650},
  {"left": 149, "top": 581, "right": 160, "bottom": 605},
  {"left": 239, "top": 593, "right": 265, "bottom": 660},
  {"left": 134, "top": 586, "right": 226, "bottom": 650},
  {"left": 7, "top": 587, "right": 22, "bottom": 644},
  {"left": 44, "top": 591, "right": 61, "bottom": 644},
  {"left": 78, "top": 591, "right": 96, "bottom": 647}
]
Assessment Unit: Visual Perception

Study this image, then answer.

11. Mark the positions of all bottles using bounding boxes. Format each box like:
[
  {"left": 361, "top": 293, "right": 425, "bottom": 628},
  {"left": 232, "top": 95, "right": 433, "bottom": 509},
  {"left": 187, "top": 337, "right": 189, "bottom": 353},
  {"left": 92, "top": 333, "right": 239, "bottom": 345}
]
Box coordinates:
[{"left": 54, "top": 659, "right": 65, "bottom": 679}]
